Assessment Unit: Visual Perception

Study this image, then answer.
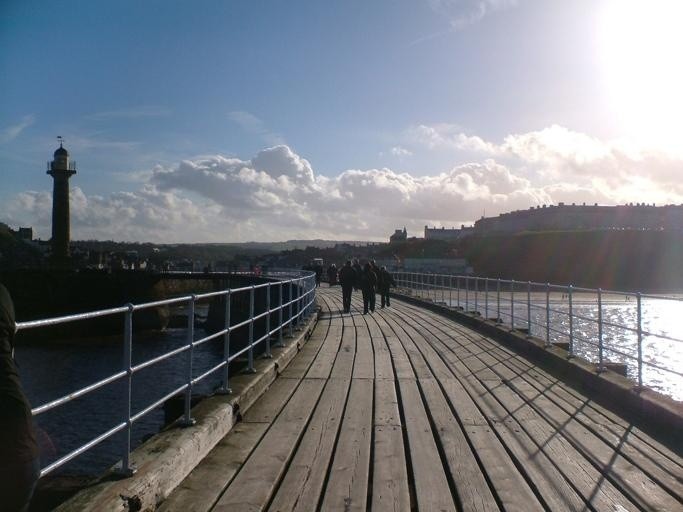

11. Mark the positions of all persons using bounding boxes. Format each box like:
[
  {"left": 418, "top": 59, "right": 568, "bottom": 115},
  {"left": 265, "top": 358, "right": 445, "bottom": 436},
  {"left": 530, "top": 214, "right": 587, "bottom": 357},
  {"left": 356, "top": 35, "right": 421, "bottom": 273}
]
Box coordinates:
[
  {"left": 124, "top": 252, "right": 269, "bottom": 276},
  {"left": 0, "top": 281, "right": 38, "bottom": 510},
  {"left": 299, "top": 257, "right": 395, "bottom": 315}
]
